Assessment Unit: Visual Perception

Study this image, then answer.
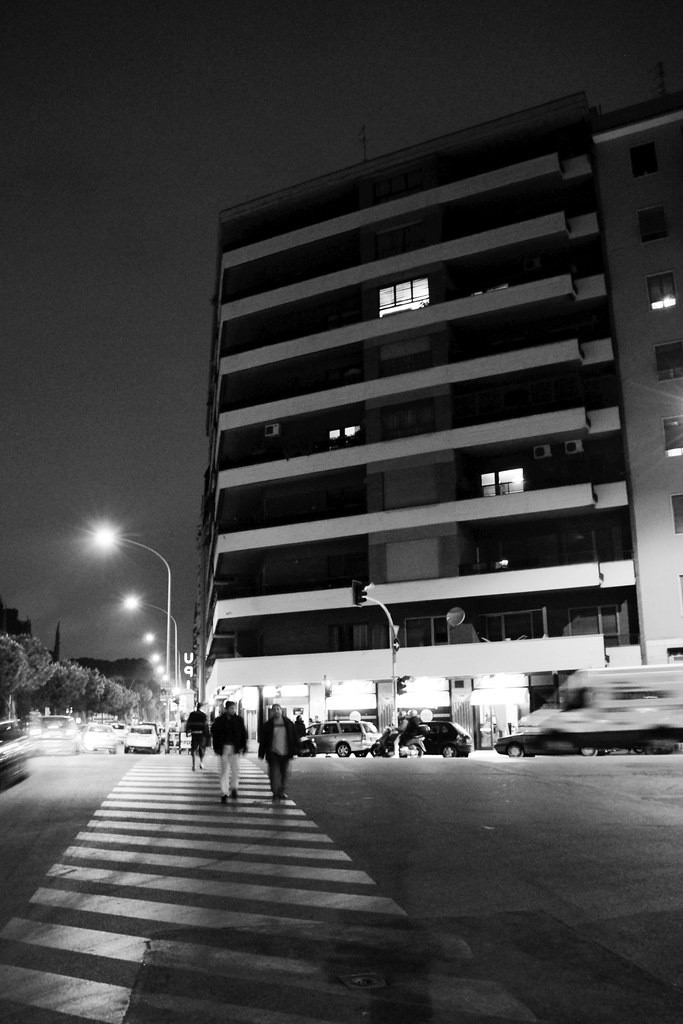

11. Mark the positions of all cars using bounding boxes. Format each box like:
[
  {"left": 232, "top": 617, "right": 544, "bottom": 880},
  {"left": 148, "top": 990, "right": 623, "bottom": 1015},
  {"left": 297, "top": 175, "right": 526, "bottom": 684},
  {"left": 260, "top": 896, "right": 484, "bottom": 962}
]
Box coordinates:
[
  {"left": 0, "top": 718, "right": 30, "bottom": 787},
  {"left": 109, "top": 723, "right": 129, "bottom": 742},
  {"left": 81, "top": 723, "right": 116, "bottom": 754},
  {"left": 494, "top": 720, "right": 617, "bottom": 757},
  {"left": 141, "top": 722, "right": 165, "bottom": 738}
]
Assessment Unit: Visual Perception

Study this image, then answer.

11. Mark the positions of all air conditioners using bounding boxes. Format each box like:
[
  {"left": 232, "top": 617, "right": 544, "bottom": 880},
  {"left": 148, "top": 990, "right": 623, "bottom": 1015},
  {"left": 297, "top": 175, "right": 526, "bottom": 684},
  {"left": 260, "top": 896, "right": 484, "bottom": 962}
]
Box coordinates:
[
  {"left": 533, "top": 445, "right": 551, "bottom": 460},
  {"left": 264, "top": 423, "right": 282, "bottom": 438},
  {"left": 565, "top": 440, "right": 583, "bottom": 454}
]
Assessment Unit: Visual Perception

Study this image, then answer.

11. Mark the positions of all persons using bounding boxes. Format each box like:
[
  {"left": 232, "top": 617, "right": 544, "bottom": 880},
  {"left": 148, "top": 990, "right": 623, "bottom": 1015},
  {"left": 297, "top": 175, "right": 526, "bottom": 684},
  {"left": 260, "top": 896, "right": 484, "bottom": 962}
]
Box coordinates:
[
  {"left": 390, "top": 710, "right": 419, "bottom": 759},
  {"left": 186, "top": 703, "right": 209, "bottom": 771},
  {"left": 309, "top": 716, "right": 321, "bottom": 725},
  {"left": 295, "top": 715, "right": 306, "bottom": 738},
  {"left": 258, "top": 704, "right": 298, "bottom": 803},
  {"left": 211, "top": 701, "right": 248, "bottom": 803}
]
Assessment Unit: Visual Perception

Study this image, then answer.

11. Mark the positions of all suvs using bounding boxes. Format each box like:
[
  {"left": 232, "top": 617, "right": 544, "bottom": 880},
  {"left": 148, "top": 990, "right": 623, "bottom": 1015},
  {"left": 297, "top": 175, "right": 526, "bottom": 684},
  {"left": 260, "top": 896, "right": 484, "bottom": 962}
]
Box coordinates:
[
  {"left": 386, "top": 720, "right": 472, "bottom": 758},
  {"left": 123, "top": 725, "right": 160, "bottom": 754},
  {"left": 34, "top": 716, "right": 81, "bottom": 756},
  {"left": 302, "top": 720, "right": 381, "bottom": 758}
]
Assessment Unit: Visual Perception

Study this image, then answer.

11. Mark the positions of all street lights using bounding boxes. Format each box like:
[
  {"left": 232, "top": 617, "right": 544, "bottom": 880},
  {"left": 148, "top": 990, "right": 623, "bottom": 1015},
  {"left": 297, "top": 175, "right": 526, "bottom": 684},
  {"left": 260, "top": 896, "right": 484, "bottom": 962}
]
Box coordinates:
[
  {"left": 146, "top": 633, "right": 181, "bottom": 712},
  {"left": 101, "top": 531, "right": 171, "bottom": 753},
  {"left": 124, "top": 598, "right": 178, "bottom": 721}
]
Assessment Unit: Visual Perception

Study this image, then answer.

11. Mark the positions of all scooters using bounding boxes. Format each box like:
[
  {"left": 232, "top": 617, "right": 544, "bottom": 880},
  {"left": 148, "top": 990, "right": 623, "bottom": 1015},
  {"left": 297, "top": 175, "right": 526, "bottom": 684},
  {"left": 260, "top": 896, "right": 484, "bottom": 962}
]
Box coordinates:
[
  {"left": 299, "top": 735, "right": 318, "bottom": 758},
  {"left": 370, "top": 724, "right": 430, "bottom": 758}
]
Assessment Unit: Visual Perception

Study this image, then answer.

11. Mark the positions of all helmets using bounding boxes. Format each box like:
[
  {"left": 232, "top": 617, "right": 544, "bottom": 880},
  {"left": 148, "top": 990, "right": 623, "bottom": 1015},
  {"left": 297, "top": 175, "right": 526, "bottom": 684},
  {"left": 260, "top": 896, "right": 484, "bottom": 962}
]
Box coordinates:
[
  {"left": 398, "top": 711, "right": 406, "bottom": 718},
  {"left": 410, "top": 710, "right": 417, "bottom": 716}
]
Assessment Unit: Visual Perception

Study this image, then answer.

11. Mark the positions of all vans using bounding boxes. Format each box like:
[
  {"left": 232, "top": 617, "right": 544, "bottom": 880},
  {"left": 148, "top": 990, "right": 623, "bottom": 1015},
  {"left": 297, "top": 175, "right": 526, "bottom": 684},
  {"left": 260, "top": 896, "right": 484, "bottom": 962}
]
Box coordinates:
[{"left": 518, "top": 667, "right": 683, "bottom": 757}]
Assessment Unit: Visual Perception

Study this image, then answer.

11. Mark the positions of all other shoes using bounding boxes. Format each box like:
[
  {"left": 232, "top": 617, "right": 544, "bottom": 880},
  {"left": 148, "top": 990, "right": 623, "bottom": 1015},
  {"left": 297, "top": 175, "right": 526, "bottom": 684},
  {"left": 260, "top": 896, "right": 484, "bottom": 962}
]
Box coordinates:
[
  {"left": 221, "top": 790, "right": 241, "bottom": 804},
  {"left": 272, "top": 792, "right": 288, "bottom": 801},
  {"left": 390, "top": 755, "right": 399, "bottom": 758},
  {"left": 191, "top": 763, "right": 205, "bottom": 771}
]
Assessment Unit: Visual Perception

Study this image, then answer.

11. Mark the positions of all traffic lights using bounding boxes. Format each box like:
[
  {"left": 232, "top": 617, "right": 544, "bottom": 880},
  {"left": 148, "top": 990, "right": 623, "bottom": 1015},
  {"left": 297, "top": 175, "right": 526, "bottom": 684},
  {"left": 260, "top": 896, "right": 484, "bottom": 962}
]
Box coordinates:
[
  {"left": 351, "top": 579, "right": 367, "bottom": 607},
  {"left": 396, "top": 677, "right": 409, "bottom": 695}
]
